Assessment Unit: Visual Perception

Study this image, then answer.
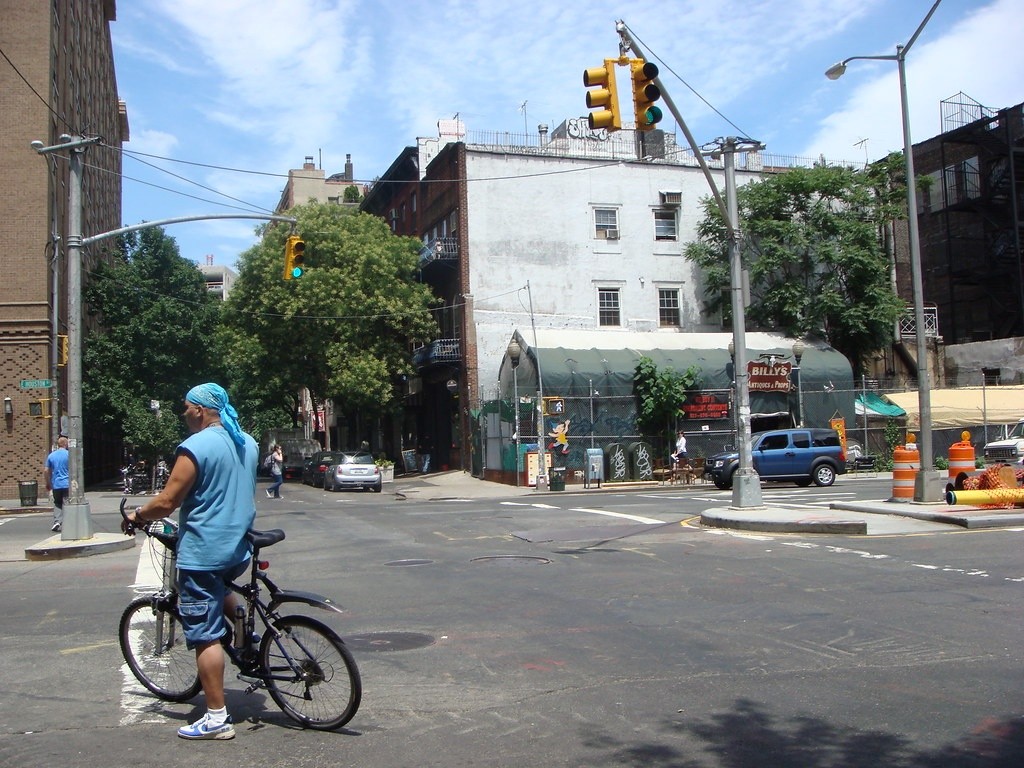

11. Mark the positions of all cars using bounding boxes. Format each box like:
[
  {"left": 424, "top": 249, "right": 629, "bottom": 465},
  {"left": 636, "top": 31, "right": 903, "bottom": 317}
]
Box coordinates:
[{"left": 258, "top": 438, "right": 383, "bottom": 493}]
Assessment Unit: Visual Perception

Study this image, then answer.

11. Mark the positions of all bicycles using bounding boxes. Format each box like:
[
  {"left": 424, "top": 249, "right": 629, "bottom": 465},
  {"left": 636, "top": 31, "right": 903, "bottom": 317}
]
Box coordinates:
[{"left": 116, "top": 498, "right": 362, "bottom": 732}]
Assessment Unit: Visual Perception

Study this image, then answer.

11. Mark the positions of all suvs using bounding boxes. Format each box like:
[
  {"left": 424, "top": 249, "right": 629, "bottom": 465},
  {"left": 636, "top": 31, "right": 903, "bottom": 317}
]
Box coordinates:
[
  {"left": 702, "top": 427, "right": 848, "bottom": 490},
  {"left": 981, "top": 416, "right": 1024, "bottom": 471}
]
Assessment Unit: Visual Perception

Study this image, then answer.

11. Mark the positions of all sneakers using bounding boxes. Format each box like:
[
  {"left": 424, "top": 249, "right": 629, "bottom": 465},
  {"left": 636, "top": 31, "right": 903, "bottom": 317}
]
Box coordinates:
[
  {"left": 178, "top": 712, "right": 236, "bottom": 740},
  {"left": 231, "top": 631, "right": 261, "bottom": 664}
]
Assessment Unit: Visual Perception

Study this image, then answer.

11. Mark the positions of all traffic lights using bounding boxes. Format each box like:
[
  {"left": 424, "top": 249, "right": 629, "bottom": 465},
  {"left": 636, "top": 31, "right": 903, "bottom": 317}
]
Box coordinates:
[
  {"left": 282, "top": 238, "right": 306, "bottom": 280},
  {"left": 549, "top": 398, "right": 565, "bottom": 414},
  {"left": 29, "top": 401, "right": 45, "bottom": 418},
  {"left": 583, "top": 58, "right": 622, "bottom": 132},
  {"left": 630, "top": 58, "right": 664, "bottom": 130}
]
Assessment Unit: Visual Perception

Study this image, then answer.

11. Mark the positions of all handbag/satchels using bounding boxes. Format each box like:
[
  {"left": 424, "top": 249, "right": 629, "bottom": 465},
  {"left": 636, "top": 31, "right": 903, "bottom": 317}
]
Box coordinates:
[{"left": 133, "top": 518, "right": 180, "bottom": 596}]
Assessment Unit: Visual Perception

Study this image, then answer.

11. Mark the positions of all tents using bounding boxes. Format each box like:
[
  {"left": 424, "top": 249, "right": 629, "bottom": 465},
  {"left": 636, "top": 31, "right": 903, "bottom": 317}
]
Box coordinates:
[{"left": 497, "top": 325, "right": 854, "bottom": 485}]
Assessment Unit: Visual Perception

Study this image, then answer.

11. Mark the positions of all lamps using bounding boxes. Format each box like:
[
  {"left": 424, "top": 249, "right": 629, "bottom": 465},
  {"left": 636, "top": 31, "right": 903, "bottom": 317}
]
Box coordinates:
[
  {"left": 447, "top": 380, "right": 459, "bottom": 399},
  {"left": 4, "top": 395, "right": 13, "bottom": 413}
]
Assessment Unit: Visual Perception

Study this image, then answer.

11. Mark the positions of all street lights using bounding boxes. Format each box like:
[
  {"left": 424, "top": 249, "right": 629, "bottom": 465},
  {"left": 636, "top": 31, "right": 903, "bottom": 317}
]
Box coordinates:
[
  {"left": 824, "top": 1, "right": 947, "bottom": 504},
  {"left": 728, "top": 337, "right": 740, "bottom": 451},
  {"left": 506, "top": 338, "right": 520, "bottom": 489},
  {"left": 29, "top": 139, "right": 60, "bottom": 506},
  {"left": 792, "top": 337, "right": 805, "bottom": 427}
]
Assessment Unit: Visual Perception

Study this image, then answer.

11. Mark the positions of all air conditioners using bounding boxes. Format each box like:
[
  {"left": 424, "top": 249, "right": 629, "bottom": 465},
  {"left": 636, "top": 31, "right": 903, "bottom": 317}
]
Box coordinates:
[
  {"left": 605, "top": 229, "right": 620, "bottom": 239},
  {"left": 663, "top": 192, "right": 682, "bottom": 205}
]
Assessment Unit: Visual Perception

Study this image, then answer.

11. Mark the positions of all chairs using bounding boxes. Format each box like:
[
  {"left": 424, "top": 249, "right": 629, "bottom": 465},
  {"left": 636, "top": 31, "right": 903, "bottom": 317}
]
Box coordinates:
[
  {"left": 670, "top": 457, "right": 690, "bottom": 484},
  {"left": 651, "top": 458, "right": 671, "bottom": 479},
  {"left": 691, "top": 457, "right": 708, "bottom": 485}
]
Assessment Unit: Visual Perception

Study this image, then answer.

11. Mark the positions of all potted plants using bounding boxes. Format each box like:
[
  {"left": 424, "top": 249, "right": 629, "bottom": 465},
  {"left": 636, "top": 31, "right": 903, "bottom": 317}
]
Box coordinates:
[{"left": 375, "top": 459, "right": 396, "bottom": 483}]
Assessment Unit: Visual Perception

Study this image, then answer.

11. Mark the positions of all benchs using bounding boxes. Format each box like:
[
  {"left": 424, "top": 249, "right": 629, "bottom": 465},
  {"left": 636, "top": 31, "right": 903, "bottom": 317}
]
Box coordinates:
[{"left": 845, "top": 455, "right": 878, "bottom": 479}]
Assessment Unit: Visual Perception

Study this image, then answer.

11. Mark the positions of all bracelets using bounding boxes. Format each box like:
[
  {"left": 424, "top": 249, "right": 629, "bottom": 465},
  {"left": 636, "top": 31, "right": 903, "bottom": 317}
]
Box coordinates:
[{"left": 134, "top": 505, "right": 147, "bottom": 523}]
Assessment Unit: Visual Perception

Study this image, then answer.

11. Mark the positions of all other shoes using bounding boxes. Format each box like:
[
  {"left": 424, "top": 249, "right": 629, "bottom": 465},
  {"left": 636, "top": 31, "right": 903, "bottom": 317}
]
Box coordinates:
[
  {"left": 51, "top": 523, "right": 60, "bottom": 531},
  {"left": 274, "top": 497, "right": 284, "bottom": 500},
  {"left": 265, "top": 489, "right": 271, "bottom": 498}
]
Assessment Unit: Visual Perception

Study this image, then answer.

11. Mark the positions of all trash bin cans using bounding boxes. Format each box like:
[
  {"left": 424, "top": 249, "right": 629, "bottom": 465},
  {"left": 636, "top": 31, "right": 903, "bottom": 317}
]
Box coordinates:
[
  {"left": 546, "top": 466, "right": 566, "bottom": 491},
  {"left": 18, "top": 479, "right": 38, "bottom": 507}
]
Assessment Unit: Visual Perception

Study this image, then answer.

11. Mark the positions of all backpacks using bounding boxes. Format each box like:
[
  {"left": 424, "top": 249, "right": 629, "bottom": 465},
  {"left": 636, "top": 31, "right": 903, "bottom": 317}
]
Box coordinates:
[{"left": 264, "top": 452, "right": 276, "bottom": 472}]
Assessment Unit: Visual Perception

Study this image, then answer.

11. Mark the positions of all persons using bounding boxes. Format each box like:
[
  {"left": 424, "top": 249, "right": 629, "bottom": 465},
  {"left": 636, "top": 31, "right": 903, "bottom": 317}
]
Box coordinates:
[
  {"left": 128, "top": 452, "right": 136, "bottom": 465},
  {"left": 265, "top": 444, "right": 284, "bottom": 499},
  {"left": 671, "top": 430, "right": 696, "bottom": 482},
  {"left": 121, "top": 382, "right": 259, "bottom": 742},
  {"left": 359, "top": 436, "right": 370, "bottom": 450},
  {"left": 43, "top": 437, "right": 71, "bottom": 532}
]
